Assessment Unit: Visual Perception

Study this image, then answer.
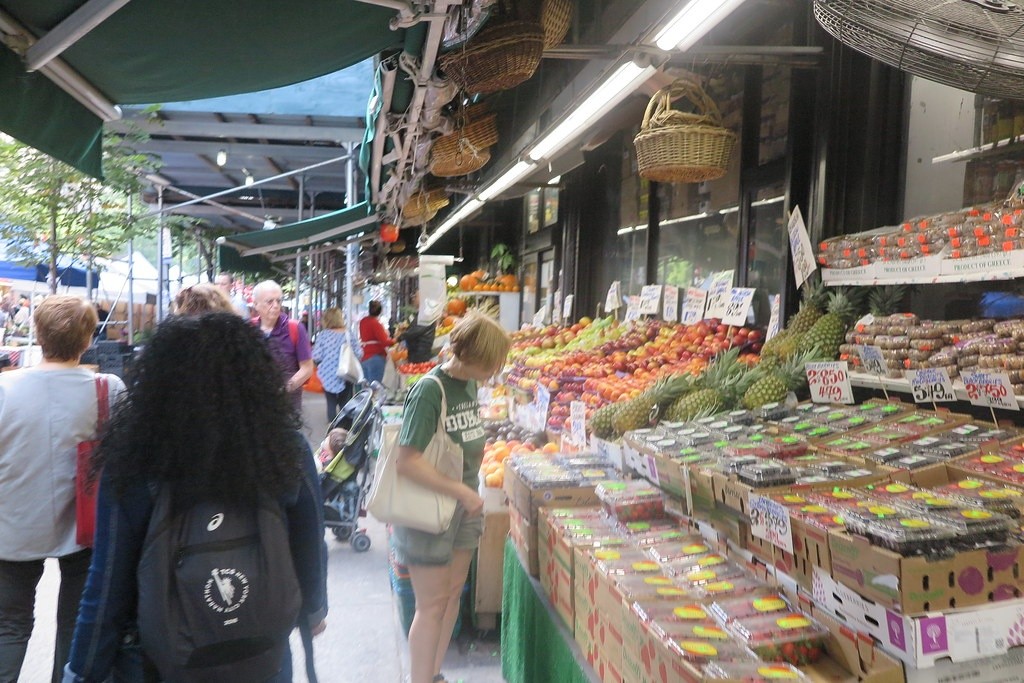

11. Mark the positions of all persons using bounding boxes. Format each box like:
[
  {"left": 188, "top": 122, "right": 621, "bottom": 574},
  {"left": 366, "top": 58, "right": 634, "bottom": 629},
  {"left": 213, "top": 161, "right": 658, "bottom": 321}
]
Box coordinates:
[
  {"left": 62, "top": 312, "right": 329, "bottom": 683},
  {"left": 392, "top": 291, "right": 437, "bottom": 363},
  {"left": 0, "top": 295, "right": 133, "bottom": 683},
  {"left": 390, "top": 313, "right": 512, "bottom": 683},
  {"left": 312, "top": 307, "right": 362, "bottom": 431},
  {"left": 360, "top": 300, "right": 397, "bottom": 389},
  {"left": 214, "top": 272, "right": 250, "bottom": 319},
  {"left": 0, "top": 291, "right": 39, "bottom": 346},
  {"left": 174, "top": 283, "right": 234, "bottom": 315},
  {"left": 314, "top": 427, "right": 350, "bottom": 471},
  {"left": 93, "top": 303, "right": 110, "bottom": 341},
  {"left": 246, "top": 280, "right": 313, "bottom": 415}
]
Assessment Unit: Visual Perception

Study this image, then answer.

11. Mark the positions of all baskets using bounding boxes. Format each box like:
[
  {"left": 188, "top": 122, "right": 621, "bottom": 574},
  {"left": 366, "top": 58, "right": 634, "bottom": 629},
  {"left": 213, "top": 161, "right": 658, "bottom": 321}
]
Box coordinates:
[
  {"left": 634, "top": 77, "right": 735, "bottom": 183},
  {"left": 403, "top": 188, "right": 449, "bottom": 226},
  {"left": 437, "top": 22, "right": 543, "bottom": 93},
  {"left": 429, "top": 99, "right": 499, "bottom": 177},
  {"left": 542, "top": 0, "right": 573, "bottom": 49}
]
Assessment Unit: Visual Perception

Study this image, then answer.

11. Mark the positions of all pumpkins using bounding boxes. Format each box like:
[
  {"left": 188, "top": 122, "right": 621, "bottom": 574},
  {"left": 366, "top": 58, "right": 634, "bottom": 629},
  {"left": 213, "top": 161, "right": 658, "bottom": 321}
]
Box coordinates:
[
  {"left": 442, "top": 269, "right": 515, "bottom": 328},
  {"left": 390, "top": 345, "right": 409, "bottom": 362}
]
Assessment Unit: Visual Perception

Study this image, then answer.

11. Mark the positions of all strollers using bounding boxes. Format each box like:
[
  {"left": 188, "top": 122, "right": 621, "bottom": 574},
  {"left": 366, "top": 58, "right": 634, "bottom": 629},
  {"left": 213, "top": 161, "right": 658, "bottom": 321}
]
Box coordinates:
[{"left": 311, "top": 386, "right": 386, "bottom": 553}]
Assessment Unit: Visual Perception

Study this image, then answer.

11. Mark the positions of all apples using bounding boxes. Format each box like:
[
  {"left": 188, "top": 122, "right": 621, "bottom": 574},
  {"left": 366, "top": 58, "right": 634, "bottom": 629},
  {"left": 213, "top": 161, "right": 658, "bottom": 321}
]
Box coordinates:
[{"left": 477, "top": 317, "right": 760, "bottom": 489}]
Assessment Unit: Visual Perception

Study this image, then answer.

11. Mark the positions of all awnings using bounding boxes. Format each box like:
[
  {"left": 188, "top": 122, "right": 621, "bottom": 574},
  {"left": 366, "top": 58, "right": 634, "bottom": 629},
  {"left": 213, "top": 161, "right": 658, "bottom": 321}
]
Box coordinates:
[{"left": 217, "top": 202, "right": 377, "bottom": 274}]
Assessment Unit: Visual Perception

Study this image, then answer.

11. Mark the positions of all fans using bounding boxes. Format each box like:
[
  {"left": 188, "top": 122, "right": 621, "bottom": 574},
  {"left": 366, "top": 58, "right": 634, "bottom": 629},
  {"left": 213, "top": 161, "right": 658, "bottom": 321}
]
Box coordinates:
[{"left": 811, "top": 0, "right": 1023, "bottom": 101}]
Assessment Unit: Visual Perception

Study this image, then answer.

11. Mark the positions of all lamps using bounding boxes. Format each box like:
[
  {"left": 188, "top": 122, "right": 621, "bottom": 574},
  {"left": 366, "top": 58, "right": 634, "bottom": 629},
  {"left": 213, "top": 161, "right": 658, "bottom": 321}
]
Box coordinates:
[
  {"left": 643, "top": 0, "right": 746, "bottom": 52},
  {"left": 417, "top": 46, "right": 672, "bottom": 254}
]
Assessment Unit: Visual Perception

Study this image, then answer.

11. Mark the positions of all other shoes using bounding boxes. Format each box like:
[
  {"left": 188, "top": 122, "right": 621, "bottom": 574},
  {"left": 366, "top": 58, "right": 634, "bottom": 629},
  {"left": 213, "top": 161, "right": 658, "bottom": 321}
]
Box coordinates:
[{"left": 433, "top": 674, "right": 448, "bottom": 683}]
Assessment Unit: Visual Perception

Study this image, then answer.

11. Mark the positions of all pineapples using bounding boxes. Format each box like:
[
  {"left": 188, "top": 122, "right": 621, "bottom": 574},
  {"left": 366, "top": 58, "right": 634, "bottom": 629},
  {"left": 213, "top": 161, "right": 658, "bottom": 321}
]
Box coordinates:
[{"left": 588, "top": 287, "right": 904, "bottom": 441}]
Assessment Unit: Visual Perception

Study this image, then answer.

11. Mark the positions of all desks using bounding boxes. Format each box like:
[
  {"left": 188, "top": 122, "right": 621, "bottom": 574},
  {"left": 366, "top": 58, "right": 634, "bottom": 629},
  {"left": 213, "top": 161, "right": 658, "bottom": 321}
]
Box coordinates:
[{"left": 501, "top": 538, "right": 604, "bottom": 683}]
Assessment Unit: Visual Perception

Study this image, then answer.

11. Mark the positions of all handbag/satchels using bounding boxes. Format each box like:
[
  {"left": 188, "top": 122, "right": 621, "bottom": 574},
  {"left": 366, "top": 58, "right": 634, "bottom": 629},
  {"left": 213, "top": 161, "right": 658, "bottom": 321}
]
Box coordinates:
[
  {"left": 302, "top": 364, "right": 323, "bottom": 394},
  {"left": 76, "top": 376, "right": 109, "bottom": 548},
  {"left": 336, "top": 330, "right": 364, "bottom": 384},
  {"left": 367, "top": 375, "right": 461, "bottom": 533}
]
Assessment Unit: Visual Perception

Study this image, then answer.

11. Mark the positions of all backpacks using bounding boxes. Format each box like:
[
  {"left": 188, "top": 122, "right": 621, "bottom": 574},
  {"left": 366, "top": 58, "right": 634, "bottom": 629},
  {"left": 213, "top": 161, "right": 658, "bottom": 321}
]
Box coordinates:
[{"left": 124, "top": 456, "right": 317, "bottom": 683}]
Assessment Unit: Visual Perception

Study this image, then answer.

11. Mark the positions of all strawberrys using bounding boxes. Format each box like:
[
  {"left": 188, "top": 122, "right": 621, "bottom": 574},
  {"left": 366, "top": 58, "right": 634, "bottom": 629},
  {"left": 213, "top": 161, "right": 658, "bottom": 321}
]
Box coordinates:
[{"left": 596, "top": 479, "right": 827, "bottom": 670}]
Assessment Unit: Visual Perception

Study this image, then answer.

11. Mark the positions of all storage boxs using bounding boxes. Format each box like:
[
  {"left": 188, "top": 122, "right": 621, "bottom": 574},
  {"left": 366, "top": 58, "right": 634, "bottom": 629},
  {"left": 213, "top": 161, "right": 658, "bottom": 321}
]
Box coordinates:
[
  {"left": 502, "top": 451, "right": 906, "bottom": 683},
  {"left": 80, "top": 341, "right": 141, "bottom": 398},
  {"left": 623, "top": 396, "right": 1024, "bottom": 683}
]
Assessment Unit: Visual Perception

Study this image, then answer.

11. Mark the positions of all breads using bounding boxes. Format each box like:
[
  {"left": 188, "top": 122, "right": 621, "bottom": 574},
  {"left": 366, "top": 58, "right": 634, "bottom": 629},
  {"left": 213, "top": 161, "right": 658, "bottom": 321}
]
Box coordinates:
[{"left": 817, "top": 194, "right": 1024, "bottom": 397}]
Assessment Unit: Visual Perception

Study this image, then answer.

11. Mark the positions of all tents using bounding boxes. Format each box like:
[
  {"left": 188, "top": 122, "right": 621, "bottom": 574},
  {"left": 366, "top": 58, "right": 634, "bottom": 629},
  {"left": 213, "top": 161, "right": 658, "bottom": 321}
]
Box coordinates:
[
  {"left": 0, "top": 222, "right": 99, "bottom": 344},
  {"left": 98, "top": 251, "right": 198, "bottom": 304}
]
